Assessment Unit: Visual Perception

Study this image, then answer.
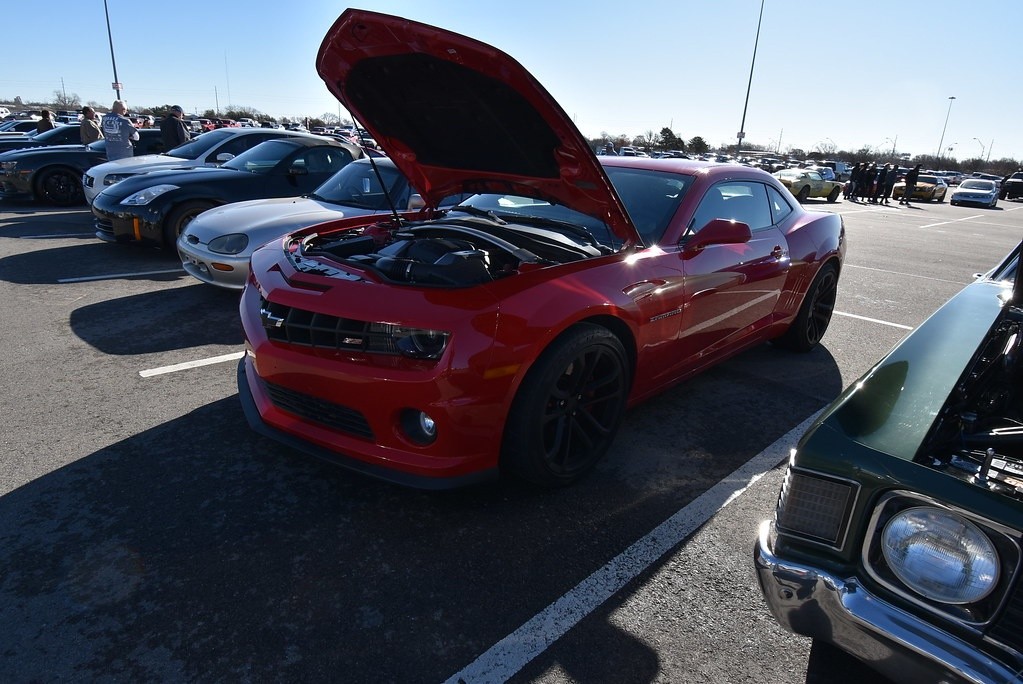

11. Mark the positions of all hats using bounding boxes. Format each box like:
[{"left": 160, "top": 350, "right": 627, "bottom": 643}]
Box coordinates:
[{"left": 171, "top": 105, "right": 183, "bottom": 113}]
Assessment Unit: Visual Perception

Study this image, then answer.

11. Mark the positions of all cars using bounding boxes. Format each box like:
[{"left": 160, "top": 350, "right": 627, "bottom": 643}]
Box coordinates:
[
  {"left": 0, "top": 102, "right": 384, "bottom": 151},
  {"left": 82, "top": 128, "right": 317, "bottom": 207},
  {"left": 91, "top": 137, "right": 385, "bottom": 255},
  {"left": 591, "top": 139, "right": 1023, "bottom": 210},
  {"left": 0, "top": 126, "right": 194, "bottom": 209},
  {"left": 235, "top": 6, "right": 849, "bottom": 503},
  {"left": 175, "top": 156, "right": 475, "bottom": 291},
  {"left": 750, "top": 239, "right": 1023, "bottom": 684},
  {"left": 0, "top": 124, "right": 90, "bottom": 153}
]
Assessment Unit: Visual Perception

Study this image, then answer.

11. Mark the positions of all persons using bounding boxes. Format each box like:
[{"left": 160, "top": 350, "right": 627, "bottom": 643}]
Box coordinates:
[
  {"left": 842, "top": 160, "right": 923, "bottom": 206},
  {"left": 36, "top": 110, "right": 56, "bottom": 135},
  {"left": 603, "top": 141, "right": 618, "bottom": 156},
  {"left": 103, "top": 100, "right": 140, "bottom": 162},
  {"left": 142, "top": 117, "right": 151, "bottom": 129},
  {"left": 80, "top": 106, "right": 106, "bottom": 145},
  {"left": 159, "top": 105, "right": 190, "bottom": 153}
]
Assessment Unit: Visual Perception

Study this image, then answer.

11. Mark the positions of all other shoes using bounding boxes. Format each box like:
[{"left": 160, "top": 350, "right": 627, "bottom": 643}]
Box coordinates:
[{"left": 844, "top": 195, "right": 910, "bottom": 206}]
